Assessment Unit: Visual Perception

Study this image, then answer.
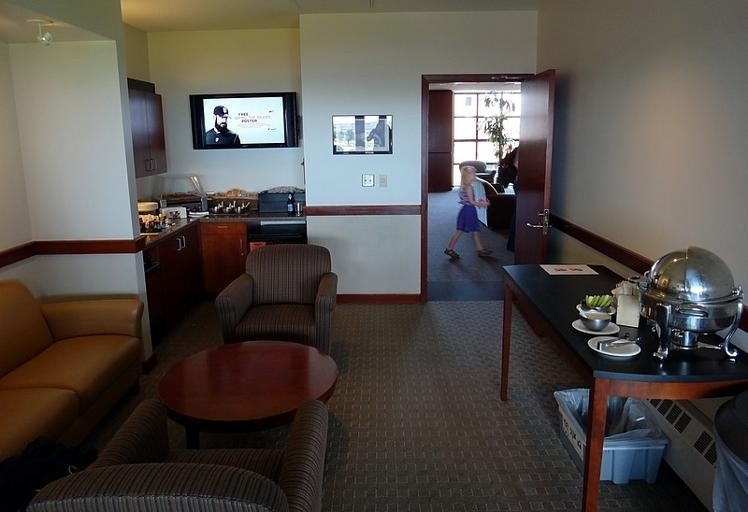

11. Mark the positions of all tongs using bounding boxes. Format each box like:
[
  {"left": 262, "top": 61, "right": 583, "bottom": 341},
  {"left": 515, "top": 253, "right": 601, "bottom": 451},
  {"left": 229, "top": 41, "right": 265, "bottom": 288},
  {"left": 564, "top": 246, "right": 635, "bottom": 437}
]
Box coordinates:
[{"left": 597, "top": 332, "right": 644, "bottom": 351}]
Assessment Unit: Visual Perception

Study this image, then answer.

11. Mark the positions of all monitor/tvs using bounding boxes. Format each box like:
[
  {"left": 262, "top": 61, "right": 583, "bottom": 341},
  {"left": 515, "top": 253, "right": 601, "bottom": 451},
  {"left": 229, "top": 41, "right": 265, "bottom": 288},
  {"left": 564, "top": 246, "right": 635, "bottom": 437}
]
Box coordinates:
[{"left": 189, "top": 92, "right": 298, "bottom": 148}]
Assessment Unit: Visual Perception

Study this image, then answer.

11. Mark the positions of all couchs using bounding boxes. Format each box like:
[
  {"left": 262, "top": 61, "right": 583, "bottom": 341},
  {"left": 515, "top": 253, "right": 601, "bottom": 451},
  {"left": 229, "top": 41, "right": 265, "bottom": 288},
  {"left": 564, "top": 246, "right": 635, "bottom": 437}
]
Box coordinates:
[{"left": 0, "top": 279, "right": 146, "bottom": 497}]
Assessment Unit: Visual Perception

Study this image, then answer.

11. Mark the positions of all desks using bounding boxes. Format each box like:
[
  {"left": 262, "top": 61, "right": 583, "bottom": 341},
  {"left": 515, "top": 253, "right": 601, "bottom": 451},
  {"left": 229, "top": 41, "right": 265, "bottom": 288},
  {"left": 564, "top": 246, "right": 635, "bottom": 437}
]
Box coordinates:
[{"left": 500, "top": 263, "right": 746, "bottom": 508}]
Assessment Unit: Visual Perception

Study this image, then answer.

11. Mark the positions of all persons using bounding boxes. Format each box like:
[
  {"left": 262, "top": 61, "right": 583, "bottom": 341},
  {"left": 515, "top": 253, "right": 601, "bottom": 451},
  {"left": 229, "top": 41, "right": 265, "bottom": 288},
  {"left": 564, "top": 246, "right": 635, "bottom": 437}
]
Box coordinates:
[
  {"left": 205, "top": 105, "right": 240, "bottom": 145},
  {"left": 506, "top": 144, "right": 519, "bottom": 253},
  {"left": 444, "top": 166, "right": 493, "bottom": 261}
]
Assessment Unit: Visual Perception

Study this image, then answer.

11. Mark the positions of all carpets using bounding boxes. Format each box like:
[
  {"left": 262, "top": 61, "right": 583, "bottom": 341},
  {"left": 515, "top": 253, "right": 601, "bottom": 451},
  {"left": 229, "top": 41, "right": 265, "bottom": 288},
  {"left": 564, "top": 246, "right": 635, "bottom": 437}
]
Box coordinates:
[
  {"left": 0, "top": 299, "right": 711, "bottom": 512},
  {"left": 428, "top": 185, "right": 514, "bottom": 283}
]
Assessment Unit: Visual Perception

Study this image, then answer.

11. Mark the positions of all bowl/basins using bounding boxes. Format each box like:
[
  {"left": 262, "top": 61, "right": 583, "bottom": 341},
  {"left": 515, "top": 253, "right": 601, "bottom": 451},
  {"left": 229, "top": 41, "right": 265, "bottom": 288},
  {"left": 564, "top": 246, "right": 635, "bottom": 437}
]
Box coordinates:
[
  {"left": 210, "top": 204, "right": 250, "bottom": 214},
  {"left": 578, "top": 312, "right": 612, "bottom": 332}
]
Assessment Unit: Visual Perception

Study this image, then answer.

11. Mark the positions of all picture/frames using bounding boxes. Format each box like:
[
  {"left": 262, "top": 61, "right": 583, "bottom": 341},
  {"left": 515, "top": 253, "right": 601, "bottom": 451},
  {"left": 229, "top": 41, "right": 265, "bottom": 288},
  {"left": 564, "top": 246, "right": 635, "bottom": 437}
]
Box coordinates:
[{"left": 331, "top": 114, "right": 393, "bottom": 154}]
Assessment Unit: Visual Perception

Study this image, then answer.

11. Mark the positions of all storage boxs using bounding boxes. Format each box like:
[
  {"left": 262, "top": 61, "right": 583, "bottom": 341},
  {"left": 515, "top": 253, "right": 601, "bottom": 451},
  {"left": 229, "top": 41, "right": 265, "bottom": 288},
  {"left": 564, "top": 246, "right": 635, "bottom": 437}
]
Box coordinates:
[{"left": 553, "top": 390, "right": 671, "bottom": 486}]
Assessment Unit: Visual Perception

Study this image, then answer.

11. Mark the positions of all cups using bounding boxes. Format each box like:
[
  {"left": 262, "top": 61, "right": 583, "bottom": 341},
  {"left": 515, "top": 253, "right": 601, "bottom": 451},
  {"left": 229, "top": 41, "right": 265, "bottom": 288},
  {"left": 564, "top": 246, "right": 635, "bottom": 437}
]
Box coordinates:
[
  {"left": 293, "top": 202, "right": 303, "bottom": 216},
  {"left": 160, "top": 200, "right": 167, "bottom": 208}
]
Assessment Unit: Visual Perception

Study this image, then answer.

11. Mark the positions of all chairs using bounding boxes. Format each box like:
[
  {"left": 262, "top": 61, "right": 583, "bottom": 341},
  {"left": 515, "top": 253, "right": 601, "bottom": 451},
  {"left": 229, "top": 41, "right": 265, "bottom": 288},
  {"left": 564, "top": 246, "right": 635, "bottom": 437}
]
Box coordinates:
[
  {"left": 461, "top": 151, "right": 517, "bottom": 229},
  {"left": 30, "top": 399, "right": 329, "bottom": 510},
  {"left": 214, "top": 243, "right": 338, "bottom": 355}
]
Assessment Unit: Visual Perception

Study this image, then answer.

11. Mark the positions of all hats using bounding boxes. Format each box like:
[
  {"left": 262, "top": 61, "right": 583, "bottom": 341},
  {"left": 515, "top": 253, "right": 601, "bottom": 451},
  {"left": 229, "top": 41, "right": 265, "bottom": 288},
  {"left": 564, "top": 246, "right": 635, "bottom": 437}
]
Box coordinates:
[{"left": 213, "top": 106, "right": 232, "bottom": 119}]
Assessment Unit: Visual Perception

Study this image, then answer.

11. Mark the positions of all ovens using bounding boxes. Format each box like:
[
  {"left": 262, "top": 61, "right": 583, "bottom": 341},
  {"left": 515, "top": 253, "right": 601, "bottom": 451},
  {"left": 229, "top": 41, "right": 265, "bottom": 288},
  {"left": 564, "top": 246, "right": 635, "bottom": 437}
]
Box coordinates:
[{"left": 244, "top": 219, "right": 307, "bottom": 253}]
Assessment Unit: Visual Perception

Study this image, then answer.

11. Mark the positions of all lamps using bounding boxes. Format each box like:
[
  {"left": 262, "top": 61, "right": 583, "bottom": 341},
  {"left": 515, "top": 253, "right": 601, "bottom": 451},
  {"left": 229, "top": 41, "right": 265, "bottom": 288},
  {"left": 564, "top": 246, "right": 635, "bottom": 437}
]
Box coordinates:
[{"left": 26, "top": 18, "right": 55, "bottom": 47}]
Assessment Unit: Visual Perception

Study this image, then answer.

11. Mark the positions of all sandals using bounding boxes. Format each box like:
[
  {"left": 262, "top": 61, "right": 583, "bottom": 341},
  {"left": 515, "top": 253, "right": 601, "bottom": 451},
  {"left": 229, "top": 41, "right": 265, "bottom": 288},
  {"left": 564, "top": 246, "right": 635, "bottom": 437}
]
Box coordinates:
[
  {"left": 443, "top": 248, "right": 459, "bottom": 259},
  {"left": 475, "top": 248, "right": 493, "bottom": 256}
]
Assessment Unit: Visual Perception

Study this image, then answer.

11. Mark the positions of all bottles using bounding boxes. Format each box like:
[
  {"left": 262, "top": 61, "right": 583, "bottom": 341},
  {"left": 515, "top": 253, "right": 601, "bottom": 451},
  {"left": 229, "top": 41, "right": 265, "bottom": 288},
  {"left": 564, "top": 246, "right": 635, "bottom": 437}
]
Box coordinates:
[
  {"left": 287, "top": 192, "right": 295, "bottom": 215},
  {"left": 139, "top": 213, "right": 170, "bottom": 234},
  {"left": 192, "top": 204, "right": 201, "bottom": 212},
  {"left": 201, "top": 195, "right": 207, "bottom": 212}
]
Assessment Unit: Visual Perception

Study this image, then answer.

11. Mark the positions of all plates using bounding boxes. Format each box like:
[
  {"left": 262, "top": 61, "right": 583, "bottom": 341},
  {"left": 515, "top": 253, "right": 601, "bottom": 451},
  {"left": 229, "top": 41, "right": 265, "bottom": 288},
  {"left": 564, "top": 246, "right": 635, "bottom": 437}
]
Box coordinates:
[
  {"left": 586, "top": 336, "right": 642, "bottom": 358},
  {"left": 188, "top": 215, "right": 206, "bottom": 218},
  {"left": 575, "top": 303, "right": 616, "bottom": 316},
  {"left": 571, "top": 319, "right": 621, "bottom": 335}
]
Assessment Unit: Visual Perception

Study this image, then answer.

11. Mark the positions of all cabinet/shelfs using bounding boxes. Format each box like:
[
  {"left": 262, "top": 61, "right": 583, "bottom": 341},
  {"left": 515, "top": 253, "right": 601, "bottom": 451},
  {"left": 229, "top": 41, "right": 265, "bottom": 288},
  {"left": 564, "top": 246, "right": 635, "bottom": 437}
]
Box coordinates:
[
  {"left": 140, "top": 220, "right": 202, "bottom": 344},
  {"left": 128, "top": 91, "right": 171, "bottom": 179},
  {"left": 199, "top": 222, "right": 247, "bottom": 294},
  {"left": 64, "top": 413, "right": 67, "bottom": 415}
]
